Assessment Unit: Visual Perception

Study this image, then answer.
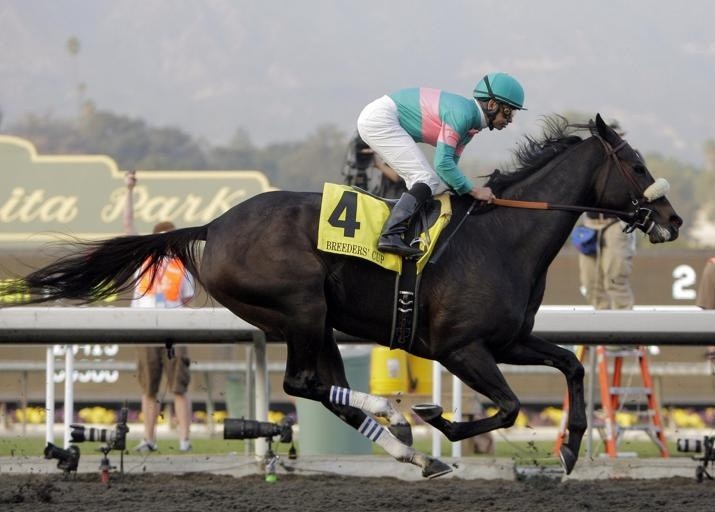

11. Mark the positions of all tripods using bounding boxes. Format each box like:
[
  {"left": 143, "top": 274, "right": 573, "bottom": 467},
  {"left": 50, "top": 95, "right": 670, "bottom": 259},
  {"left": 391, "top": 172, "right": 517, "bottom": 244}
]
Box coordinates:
[{"left": 255, "top": 437, "right": 295, "bottom": 473}]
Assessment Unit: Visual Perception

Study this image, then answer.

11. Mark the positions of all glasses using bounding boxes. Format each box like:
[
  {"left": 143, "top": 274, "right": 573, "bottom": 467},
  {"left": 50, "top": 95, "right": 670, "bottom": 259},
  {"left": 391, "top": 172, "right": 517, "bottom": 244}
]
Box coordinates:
[{"left": 498, "top": 102, "right": 516, "bottom": 117}]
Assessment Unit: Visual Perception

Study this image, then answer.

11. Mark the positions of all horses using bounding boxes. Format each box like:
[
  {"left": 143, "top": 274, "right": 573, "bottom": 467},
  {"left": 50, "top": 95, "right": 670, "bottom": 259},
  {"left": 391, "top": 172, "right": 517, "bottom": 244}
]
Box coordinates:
[{"left": 0, "top": 112, "right": 684, "bottom": 481}]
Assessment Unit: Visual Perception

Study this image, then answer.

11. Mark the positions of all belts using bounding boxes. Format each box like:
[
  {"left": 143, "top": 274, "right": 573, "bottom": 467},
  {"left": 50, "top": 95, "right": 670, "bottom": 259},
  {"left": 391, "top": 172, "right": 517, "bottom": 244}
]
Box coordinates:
[{"left": 586, "top": 211, "right": 617, "bottom": 219}]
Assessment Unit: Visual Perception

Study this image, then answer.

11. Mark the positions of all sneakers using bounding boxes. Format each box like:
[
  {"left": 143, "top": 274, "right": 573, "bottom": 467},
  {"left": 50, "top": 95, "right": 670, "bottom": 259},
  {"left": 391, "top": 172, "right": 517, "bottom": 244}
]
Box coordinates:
[
  {"left": 131, "top": 439, "right": 158, "bottom": 453},
  {"left": 179, "top": 440, "right": 192, "bottom": 452}
]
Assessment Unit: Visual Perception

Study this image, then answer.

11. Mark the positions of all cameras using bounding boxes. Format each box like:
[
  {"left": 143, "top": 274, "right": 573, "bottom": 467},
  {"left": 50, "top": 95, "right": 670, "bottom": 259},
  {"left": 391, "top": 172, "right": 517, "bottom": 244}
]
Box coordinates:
[
  {"left": 223, "top": 417, "right": 292, "bottom": 443},
  {"left": 677, "top": 435, "right": 715, "bottom": 467},
  {"left": 43, "top": 442, "right": 80, "bottom": 471},
  {"left": 69, "top": 407, "right": 130, "bottom": 450}
]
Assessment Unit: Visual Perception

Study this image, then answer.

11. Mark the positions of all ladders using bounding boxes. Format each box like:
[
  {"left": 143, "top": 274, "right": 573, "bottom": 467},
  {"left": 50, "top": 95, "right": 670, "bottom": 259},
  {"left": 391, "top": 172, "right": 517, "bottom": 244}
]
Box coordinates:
[{"left": 553, "top": 343, "right": 670, "bottom": 458}]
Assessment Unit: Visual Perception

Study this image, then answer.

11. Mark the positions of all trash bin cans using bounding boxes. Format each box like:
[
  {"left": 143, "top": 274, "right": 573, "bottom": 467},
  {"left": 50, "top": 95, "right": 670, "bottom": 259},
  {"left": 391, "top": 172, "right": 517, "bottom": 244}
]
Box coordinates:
[
  {"left": 225, "top": 377, "right": 254, "bottom": 421},
  {"left": 296, "top": 355, "right": 373, "bottom": 456}
]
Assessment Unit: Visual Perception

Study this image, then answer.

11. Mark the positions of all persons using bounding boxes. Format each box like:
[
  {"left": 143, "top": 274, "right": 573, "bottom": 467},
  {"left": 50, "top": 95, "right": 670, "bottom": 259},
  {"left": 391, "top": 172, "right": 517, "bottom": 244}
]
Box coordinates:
[
  {"left": 357, "top": 73, "right": 527, "bottom": 256},
  {"left": 372, "top": 153, "right": 408, "bottom": 199},
  {"left": 131, "top": 223, "right": 193, "bottom": 453},
  {"left": 580, "top": 212, "right": 636, "bottom": 350}
]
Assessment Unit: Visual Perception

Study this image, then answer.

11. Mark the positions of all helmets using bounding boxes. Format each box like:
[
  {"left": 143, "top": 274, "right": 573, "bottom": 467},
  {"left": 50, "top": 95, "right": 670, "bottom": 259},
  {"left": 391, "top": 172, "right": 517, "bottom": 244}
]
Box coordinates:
[{"left": 473, "top": 72, "right": 528, "bottom": 111}]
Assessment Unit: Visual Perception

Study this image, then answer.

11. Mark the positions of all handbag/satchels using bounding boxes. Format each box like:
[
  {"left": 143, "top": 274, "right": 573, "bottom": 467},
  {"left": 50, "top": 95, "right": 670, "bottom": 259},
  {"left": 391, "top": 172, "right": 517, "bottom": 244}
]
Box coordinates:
[{"left": 571, "top": 224, "right": 604, "bottom": 256}]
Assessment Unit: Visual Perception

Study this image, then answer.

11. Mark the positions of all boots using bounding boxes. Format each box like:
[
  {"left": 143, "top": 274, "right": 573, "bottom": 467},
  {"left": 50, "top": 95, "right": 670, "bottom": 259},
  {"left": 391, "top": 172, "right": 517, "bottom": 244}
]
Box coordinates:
[{"left": 376, "top": 192, "right": 425, "bottom": 259}]
